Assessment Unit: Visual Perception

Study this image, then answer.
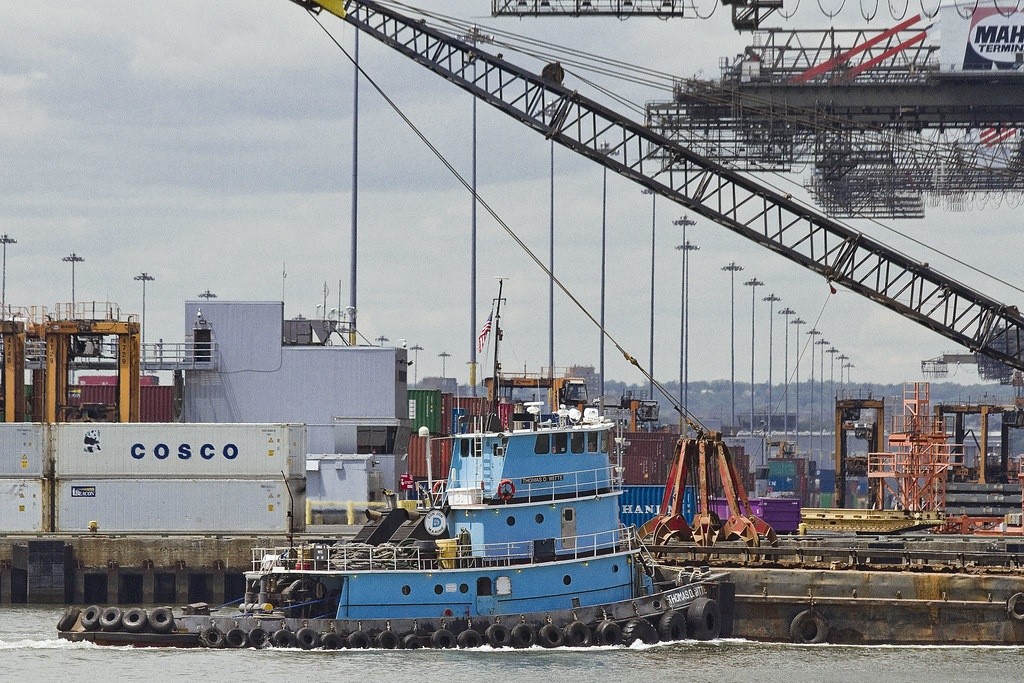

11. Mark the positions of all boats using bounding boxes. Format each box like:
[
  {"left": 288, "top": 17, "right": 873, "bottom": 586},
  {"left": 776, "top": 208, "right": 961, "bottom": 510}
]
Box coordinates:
[
  {"left": 55, "top": 408, "right": 722, "bottom": 652},
  {"left": 0, "top": 294, "right": 410, "bottom": 610}
]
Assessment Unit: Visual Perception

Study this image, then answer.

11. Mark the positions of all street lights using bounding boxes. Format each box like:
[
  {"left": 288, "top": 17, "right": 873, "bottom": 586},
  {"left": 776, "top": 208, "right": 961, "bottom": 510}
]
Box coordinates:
[
  {"left": 61, "top": 253, "right": 85, "bottom": 316},
  {"left": 533, "top": 100, "right": 567, "bottom": 434},
  {"left": 134, "top": 272, "right": 155, "bottom": 378},
  {"left": 674, "top": 211, "right": 700, "bottom": 438},
  {"left": 0, "top": 233, "right": 19, "bottom": 321},
  {"left": 593, "top": 142, "right": 623, "bottom": 424},
  {"left": 452, "top": 24, "right": 493, "bottom": 399},
  {"left": 722, "top": 257, "right": 746, "bottom": 461},
  {"left": 641, "top": 181, "right": 665, "bottom": 429},
  {"left": 743, "top": 278, "right": 856, "bottom": 473}
]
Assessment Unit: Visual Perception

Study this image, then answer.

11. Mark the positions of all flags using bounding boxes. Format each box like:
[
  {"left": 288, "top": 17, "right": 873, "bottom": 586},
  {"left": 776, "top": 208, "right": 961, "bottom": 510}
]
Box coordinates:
[{"left": 479, "top": 311, "right": 493, "bottom": 353}]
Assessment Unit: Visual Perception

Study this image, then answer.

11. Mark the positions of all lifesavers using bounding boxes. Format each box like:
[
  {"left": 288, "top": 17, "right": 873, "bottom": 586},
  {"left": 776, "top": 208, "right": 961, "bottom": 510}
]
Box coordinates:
[
  {"left": 433, "top": 481, "right": 447, "bottom": 500},
  {"left": 485, "top": 621, "right": 621, "bottom": 648},
  {"left": 457, "top": 629, "right": 483, "bottom": 649},
  {"left": 789, "top": 609, "right": 830, "bottom": 644},
  {"left": 1008, "top": 592, "right": 1024, "bottom": 620},
  {"left": 320, "top": 632, "right": 343, "bottom": 650},
  {"left": 430, "top": 628, "right": 457, "bottom": 649},
  {"left": 623, "top": 597, "right": 723, "bottom": 649},
  {"left": 273, "top": 629, "right": 295, "bottom": 648},
  {"left": 497, "top": 479, "right": 516, "bottom": 500},
  {"left": 226, "top": 628, "right": 247, "bottom": 648},
  {"left": 81, "top": 604, "right": 175, "bottom": 633},
  {"left": 248, "top": 627, "right": 269, "bottom": 649},
  {"left": 56, "top": 605, "right": 80, "bottom": 631},
  {"left": 402, "top": 634, "right": 425, "bottom": 649},
  {"left": 296, "top": 627, "right": 319, "bottom": 650},
  {"left": 202, "top": 626, "right": 225, "bottom": 648},
  {"left": 376, "top": 630, "right": 401, "bottom": 649},
  {"left": 346, "top": 630, "right": 373, "bottom": 649}
]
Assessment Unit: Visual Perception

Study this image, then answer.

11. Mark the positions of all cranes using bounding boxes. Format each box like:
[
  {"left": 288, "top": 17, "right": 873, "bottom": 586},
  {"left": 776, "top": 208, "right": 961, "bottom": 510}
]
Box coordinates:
[{"left": 290, "top": 0, "right": 1024, "bottom": 649}]
